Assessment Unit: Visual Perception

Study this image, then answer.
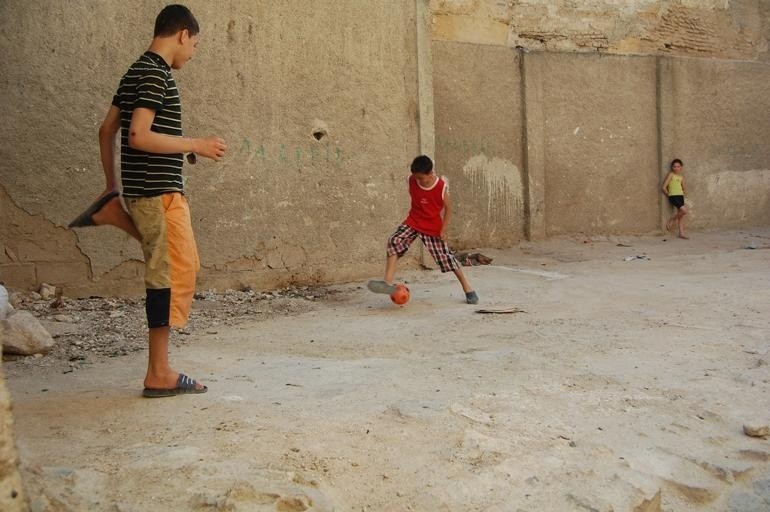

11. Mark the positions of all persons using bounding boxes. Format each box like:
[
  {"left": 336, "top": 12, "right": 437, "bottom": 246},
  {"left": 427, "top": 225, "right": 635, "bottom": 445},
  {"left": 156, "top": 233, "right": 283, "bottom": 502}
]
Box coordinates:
[
  {"left": 67, "top": 4, "right": 227, "bottom": 397},
  {"left": 364, "top": 154, "right": 481, "bottom": 303},
  {"left": 662, "top": 159, "right": 690, "bottom": 239}
]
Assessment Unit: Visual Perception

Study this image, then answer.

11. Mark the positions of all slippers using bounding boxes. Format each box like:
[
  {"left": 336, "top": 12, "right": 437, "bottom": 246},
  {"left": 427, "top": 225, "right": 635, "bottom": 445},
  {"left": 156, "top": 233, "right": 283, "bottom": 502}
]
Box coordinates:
[
  {"left": 367, "top": 280, "right": 394, "bottom": 294},
  {"left": 68, "top": 191, "right": 120, "bottom": 227},
  {"left": 143, "top": 373, "right": 208, "bottom": 397},
  {"left": 466, "top": 291, "right": 478, "bottom": 304}
]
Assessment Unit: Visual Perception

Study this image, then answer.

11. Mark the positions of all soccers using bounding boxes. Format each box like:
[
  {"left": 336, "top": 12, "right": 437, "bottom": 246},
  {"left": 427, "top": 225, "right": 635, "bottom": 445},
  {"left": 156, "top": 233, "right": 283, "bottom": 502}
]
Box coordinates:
[{"left": 390, "top": 284, "right": 410, "bottom": 305}]
